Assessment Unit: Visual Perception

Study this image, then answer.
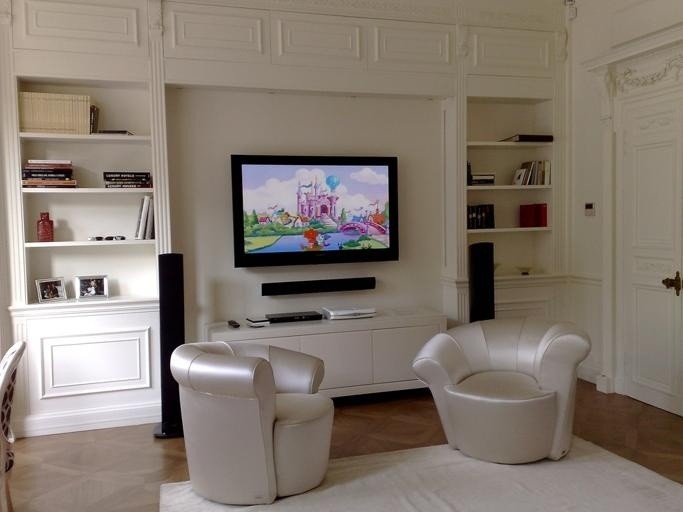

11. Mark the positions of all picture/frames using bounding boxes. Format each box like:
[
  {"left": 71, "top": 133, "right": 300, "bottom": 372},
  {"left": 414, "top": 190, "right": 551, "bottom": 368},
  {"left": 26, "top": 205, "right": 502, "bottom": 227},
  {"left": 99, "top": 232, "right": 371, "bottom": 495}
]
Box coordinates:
[
  {"left": 35, "top": 277, "right": 68, "bottom": 304},
  {"left": 74, "top": 275, "right": 109, "bottom": 300}
]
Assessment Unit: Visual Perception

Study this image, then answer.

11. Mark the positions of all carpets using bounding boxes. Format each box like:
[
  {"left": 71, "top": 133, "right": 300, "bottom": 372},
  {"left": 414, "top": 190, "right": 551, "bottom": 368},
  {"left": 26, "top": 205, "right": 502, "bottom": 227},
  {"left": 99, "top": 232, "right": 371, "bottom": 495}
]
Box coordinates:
[{"left": 159, "top": 434, "right": 683, "bottom": 512}]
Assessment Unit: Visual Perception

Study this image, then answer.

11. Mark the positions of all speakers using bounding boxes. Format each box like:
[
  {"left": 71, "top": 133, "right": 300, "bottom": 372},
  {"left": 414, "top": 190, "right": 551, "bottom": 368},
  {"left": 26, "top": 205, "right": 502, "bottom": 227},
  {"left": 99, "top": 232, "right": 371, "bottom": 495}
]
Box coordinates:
[
  {"left": 469, "top": 242, "right": 495, "bottom": 323},
  {"left": 262, "top": 277, "right": 375, "bottom": 296},
  {"left": 153, "top": 253, "right": 185, "bottom": 438}
]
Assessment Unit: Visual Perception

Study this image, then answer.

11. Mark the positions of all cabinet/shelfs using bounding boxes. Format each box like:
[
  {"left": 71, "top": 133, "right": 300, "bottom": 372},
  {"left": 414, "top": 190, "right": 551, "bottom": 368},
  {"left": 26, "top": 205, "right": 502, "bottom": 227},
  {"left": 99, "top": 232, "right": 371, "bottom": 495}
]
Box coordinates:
[{"left": 208, "top": 310, "right": 448, "bottom": 399}]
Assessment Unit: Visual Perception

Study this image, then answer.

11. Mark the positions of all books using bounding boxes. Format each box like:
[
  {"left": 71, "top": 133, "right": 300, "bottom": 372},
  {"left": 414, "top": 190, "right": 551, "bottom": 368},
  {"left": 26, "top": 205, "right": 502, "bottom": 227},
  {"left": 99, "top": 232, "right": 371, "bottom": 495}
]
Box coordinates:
[
  {"left": 511, "top": 160, "right": 551, "bottom": 186},
  {"left": 90, "top": 104, "right": 134, "bottom": 136},
  {"left": 102, "top": 171, "right": 152, "bottom": 188},
  {"left": 498, "top": 133, "right": 554, "bottom": 143},
  {"left": 134, "top": 195, "right": 154, "bottom": 241},
  {"left": 467, "top": 159, "right": 495, "bottom": 186},
  {"left": 466, "top": 203, "right": 495, "bottom": 230},
  {"left": 22, "top": 159, "right": 77, "bottom": 188},
  {"left": 519, "top": 203, "right": 547, "bottom": 227}
]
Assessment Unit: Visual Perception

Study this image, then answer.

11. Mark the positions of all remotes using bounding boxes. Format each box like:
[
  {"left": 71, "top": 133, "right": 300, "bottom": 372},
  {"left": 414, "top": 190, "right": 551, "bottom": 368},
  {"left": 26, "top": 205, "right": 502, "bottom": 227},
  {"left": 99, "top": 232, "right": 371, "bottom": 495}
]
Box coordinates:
[{"left": 228, "top": 321, "right": 240, "bottom": 328}]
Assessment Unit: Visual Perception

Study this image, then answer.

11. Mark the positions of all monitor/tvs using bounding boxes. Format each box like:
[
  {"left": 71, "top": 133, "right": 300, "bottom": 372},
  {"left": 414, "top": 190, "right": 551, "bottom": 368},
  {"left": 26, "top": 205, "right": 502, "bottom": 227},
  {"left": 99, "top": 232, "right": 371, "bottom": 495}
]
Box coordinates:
[{"left": 231, "top": 155, "right": 399, "bottom": 268}]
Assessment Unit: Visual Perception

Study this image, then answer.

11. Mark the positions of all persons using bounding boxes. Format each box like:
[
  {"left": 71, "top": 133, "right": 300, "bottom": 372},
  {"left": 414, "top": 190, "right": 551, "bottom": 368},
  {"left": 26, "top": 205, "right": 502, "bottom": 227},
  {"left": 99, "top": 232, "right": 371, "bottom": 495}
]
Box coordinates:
[
  {"left": 46, "top": 283, "right": 59, "bottom": 299},
  {"left": 90, "top": 279, "right": 97, "bottom": 289}
]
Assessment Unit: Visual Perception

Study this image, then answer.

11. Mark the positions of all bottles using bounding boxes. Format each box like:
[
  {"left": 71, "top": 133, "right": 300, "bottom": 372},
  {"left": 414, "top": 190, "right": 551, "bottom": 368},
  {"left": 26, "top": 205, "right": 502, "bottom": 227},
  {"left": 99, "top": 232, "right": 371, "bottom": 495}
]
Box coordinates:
[{"left": 36, "top": 212, "right": 53, "bottom": 242}]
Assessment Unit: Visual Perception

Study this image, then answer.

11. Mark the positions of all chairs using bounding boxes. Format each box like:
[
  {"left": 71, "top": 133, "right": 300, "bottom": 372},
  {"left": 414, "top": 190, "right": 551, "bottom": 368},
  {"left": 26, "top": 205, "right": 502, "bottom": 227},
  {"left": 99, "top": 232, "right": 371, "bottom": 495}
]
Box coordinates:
[
  {"left": 0, "top": 341, "right": 26, "bottom": 512},
  {"left": 412, "top": 316, "right": 591, "bottom": 465},
  {"left": 170, "top": 340, "right": 335, "bottom": 506}
]
